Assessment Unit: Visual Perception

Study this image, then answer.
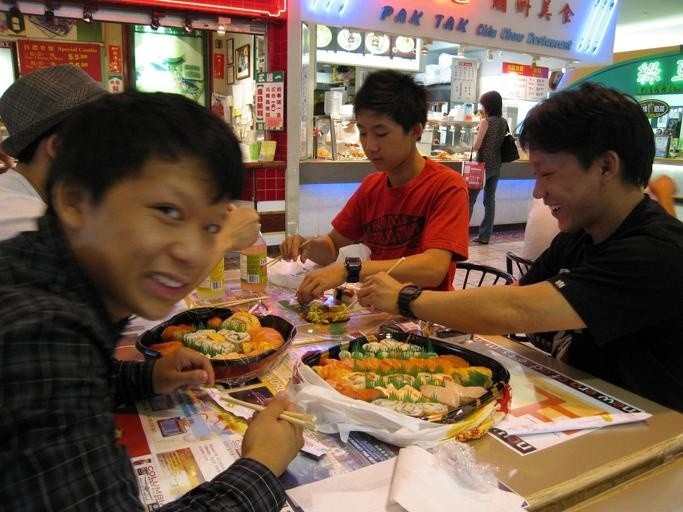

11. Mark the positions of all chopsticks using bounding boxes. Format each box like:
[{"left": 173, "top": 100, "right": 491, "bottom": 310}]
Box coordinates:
[
  {"left": 196, "top": 295, "right": 270, "bottom": 308},
  {"left": 337, "top": 257, "right": 405, "bottom": 316},
  {"left": 261, "top": 239, "right": 310, "bottom": 268},
  {"left": 218, "top": 395, "right": 317, "bottom": 426}
]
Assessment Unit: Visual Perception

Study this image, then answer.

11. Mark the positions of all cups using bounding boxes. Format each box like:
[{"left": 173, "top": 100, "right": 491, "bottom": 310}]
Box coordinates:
[
  {"left": 333, "top": 285, "right": 356, "bottom": 307},
  {"left": 248, "top": 140, "right": 262, "bottom": 162}
]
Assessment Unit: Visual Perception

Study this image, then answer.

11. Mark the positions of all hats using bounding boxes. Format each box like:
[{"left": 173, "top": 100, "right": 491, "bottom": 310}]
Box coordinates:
[{"left": 1, "top": 65, "right": 113, "bottom": 159}]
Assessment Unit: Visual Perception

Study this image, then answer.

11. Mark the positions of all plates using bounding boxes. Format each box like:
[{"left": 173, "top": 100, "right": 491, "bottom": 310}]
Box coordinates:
[
  {"left": 303, "top": 332, "right": 510, "bottom": 425},
  {"left": 323, "top": 91, "right": 343, "bottom": 116}
]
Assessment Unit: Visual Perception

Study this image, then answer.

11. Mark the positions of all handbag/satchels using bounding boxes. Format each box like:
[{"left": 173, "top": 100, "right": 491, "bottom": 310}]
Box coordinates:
[
  {"left": 461, "top": 148, "right": 486, "bottom": 190},
  {"left": 502, "top": 123, "right": 520, "bottom": 163}
]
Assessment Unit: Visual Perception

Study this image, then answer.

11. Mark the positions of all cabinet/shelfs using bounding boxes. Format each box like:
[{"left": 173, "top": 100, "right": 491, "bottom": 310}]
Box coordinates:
[{"left": 312, "top": 113, "right": 480, "bottom": 162}]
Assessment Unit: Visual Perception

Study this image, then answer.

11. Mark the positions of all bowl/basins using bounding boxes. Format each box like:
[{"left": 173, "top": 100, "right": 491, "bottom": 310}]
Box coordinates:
[{"left": 134, "top": 304, "right": 297, "bottom": 382}]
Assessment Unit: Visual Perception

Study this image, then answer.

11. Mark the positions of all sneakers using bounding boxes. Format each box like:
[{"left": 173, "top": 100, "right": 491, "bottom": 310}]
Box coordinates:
[{"left": 472, "top": 237, "right": 489, "bottom": 244}]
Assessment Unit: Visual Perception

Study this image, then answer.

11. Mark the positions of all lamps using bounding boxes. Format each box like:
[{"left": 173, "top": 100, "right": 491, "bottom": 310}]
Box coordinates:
[
  {"left": 0, "top": 1, "right": 244, "bottom": 40},
  {"left": 412, "top": 34, "right": 541, "bottom": 72}
]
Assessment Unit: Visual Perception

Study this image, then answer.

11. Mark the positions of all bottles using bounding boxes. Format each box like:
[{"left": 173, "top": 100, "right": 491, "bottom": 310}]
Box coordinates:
[
  {"left": 196, "top": 255, "right": 225, "bottom": 299},
  {"left": 464, "top": 104, "right": 472, "bottom": 123},
  {"left": 239, "top": 224, "right": 267, "bottom": 291}
]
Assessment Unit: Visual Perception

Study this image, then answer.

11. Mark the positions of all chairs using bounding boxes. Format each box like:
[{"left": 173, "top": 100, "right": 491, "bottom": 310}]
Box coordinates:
[
  {"left": 503, "top": 249, "right": 544, "bottom": 349},
  {"left": 448, "top": 257, "right": 516, "bottom": 345}
]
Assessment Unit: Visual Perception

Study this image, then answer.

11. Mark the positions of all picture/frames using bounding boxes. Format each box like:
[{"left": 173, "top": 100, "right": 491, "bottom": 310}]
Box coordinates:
[
  {"left": 234, "top": 42, "right": 249, "bottom": 81},
  {"left": 224, "top": 36, "right": 235, "bottom": 66},
  {"left": 225, "top": 65, "right": 234, "bottom": 85}
]
